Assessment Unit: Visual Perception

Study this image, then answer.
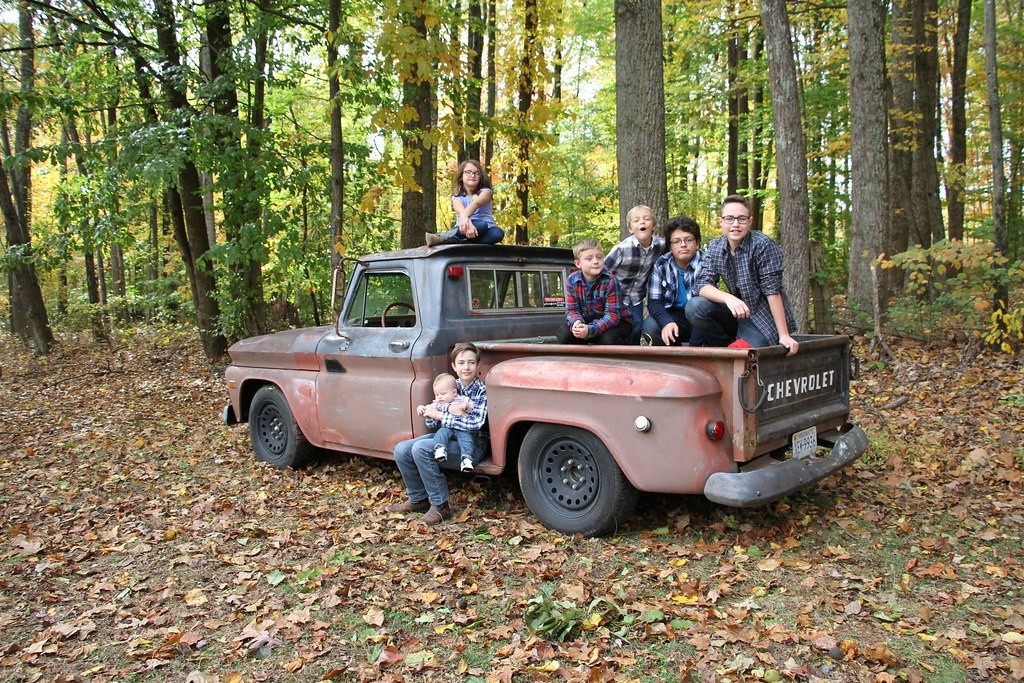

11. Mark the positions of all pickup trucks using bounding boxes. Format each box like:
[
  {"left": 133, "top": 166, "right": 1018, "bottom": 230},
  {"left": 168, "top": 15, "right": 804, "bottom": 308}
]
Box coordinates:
[{"left": 221, "top": 244, "right": 867, "bottom": 539}]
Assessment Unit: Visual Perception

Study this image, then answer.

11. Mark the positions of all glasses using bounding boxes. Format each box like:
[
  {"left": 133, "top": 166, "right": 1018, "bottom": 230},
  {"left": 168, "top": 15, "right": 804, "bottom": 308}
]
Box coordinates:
[
  {"left": 463, "top": 170, "right": 480, "bottom": 177},
  {"left": 670, "top": 236, "right": 695, "bottom": 245},
  {"left": 722, "top": 215, "right": 750, "bottom": 224}
]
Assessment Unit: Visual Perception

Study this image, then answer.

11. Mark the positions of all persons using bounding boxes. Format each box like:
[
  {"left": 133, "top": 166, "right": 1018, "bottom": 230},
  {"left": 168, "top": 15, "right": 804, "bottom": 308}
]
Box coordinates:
[
  {"left": 558, "top": 195, "right": 799, "bottom": 356},
  {"left": 384, "top": 343, "right": 488, "bottom": 525},
  {"left": 426, "top": 159, "right": 504, "bottom": 247}
]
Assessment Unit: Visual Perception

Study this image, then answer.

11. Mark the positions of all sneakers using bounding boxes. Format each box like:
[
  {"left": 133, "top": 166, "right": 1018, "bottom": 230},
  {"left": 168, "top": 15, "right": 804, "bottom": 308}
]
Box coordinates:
[
  {"left": 434, "top": 446, "right": 447, "bottom": 463},
  {"left": 460, "top": 457, "right": 474, "bottom": 472},
  {"left": 425, "top": 232, "right": 443, "bottom": 247}
]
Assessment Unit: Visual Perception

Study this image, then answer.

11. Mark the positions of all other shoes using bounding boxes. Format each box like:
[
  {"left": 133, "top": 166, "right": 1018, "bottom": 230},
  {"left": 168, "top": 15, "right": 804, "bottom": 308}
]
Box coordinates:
[
  {"left": 388, "top": 499, "right": 430, "bottom": 512},
  {"left": 416, "top": 505, "right": 452, "bottom": 526}
]
